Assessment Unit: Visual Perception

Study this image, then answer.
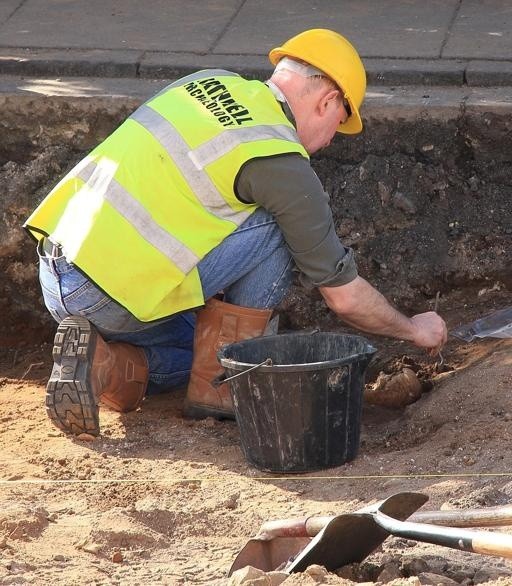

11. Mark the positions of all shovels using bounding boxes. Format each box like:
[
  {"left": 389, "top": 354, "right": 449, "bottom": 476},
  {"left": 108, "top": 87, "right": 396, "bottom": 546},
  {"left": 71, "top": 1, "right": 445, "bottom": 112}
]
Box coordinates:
[{"left": 227, "top": 492, "right": 512, "bottom": 573}]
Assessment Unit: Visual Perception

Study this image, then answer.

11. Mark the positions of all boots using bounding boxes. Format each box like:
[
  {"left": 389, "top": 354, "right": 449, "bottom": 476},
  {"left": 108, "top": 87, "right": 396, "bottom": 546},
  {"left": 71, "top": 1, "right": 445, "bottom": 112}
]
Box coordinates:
[
  {"left": 183, "top": 298, "right": 272, "bottom": 420},
  {"left": 46, "top": 316, "right": 148, "bottom": 440}
]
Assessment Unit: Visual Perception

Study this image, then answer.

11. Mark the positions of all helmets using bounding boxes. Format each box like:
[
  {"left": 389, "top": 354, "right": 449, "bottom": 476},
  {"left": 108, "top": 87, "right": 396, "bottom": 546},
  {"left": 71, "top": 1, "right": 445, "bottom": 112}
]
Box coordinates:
[{"left": 269, "top": 28, "right": 367, "bottom": 135}]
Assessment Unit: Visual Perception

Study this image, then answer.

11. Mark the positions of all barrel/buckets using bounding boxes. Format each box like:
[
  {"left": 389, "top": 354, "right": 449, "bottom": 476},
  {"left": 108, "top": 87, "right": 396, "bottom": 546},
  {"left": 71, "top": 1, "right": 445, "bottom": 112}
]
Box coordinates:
[{"left": 212, "top": 330, "right": 377, "bottom": 475}]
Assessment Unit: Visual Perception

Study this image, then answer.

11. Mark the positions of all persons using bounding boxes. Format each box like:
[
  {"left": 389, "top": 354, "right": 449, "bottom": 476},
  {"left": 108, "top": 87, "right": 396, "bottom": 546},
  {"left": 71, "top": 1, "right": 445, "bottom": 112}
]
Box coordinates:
[{"left": 21, "top": 27, "right": 449, "bottom": 437}]
